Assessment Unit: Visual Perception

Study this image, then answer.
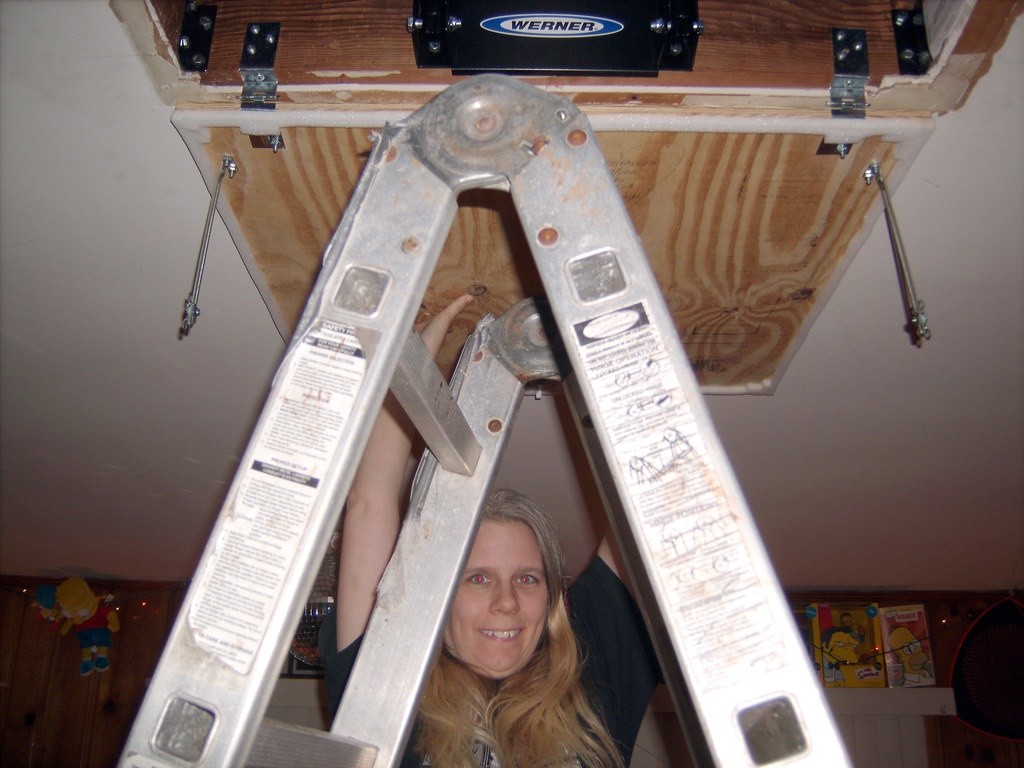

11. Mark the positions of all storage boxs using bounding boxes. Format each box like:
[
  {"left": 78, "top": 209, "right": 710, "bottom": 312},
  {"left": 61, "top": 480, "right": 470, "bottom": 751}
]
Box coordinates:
[{"left": 810, "top": 603, "right": 936, "bottom": 688}]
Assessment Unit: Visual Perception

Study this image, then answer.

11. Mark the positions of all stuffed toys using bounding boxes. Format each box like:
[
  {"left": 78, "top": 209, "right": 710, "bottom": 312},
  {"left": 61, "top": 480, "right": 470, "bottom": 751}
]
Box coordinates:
[{"left": 32, "top": 578, "right": 119, "bottom": 675}]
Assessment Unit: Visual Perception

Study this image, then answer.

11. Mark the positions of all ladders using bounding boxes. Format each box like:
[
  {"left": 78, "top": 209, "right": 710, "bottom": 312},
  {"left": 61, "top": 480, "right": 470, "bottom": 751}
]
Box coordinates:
[{"left": 113, "top": 73, "right": 853, "bottom": 768}]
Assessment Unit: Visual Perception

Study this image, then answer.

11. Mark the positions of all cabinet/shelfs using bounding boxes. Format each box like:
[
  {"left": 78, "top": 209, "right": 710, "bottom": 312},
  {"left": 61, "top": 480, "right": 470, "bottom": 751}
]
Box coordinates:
[
  {"left": 787, "top": 591, "right": 1024, "bottom": 768},
  {"left": 0, "top": 575, "right": 185, "bottom": 768}
]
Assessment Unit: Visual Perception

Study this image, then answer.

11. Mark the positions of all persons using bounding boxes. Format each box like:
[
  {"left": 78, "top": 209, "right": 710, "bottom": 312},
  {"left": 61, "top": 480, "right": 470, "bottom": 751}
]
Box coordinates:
[
  {"left": 317, "top": 295, "right": 662, "bottom": 768},
  {"left": 840, "top": 612, "right": 859, "bottom": 644}
]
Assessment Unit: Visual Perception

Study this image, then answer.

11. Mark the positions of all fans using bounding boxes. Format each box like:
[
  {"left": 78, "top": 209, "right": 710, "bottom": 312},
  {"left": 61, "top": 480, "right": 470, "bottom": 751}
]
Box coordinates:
[{"left": 948, "top": 596, "right": 1024, "bottom": 743}]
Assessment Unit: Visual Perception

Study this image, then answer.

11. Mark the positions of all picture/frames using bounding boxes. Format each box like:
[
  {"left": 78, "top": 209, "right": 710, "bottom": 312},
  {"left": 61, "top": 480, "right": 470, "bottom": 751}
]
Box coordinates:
[{"left": 290, "top": 656, "right": 324, "bottom": 678}]
